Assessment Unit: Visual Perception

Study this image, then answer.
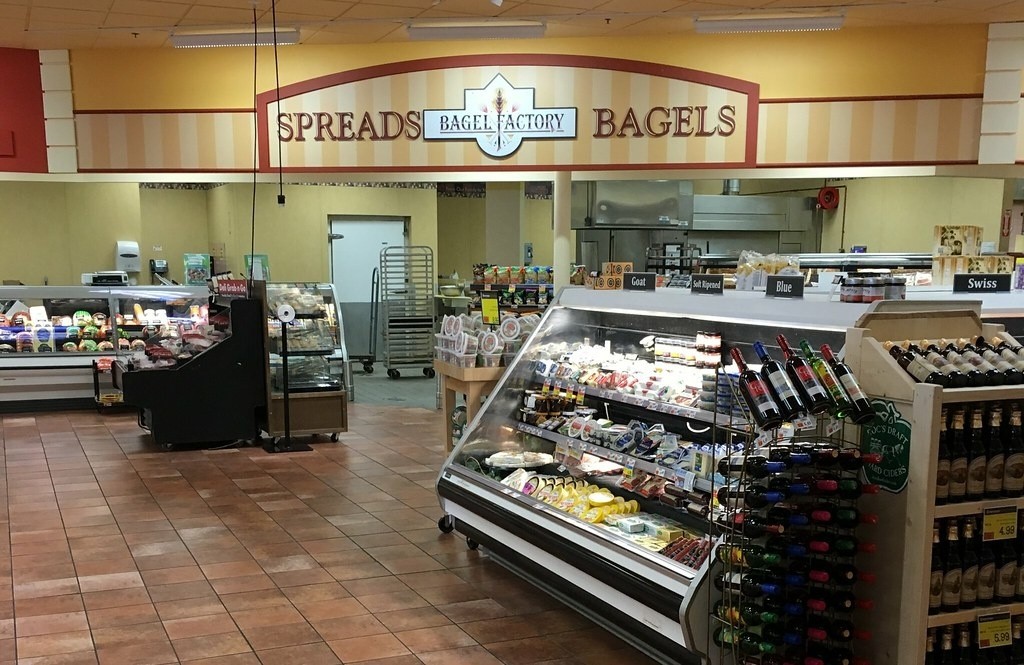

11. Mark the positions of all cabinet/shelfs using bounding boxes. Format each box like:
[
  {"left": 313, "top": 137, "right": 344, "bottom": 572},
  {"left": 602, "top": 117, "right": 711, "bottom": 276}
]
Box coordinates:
[
  {"left": 267, "top": 302, "right": 343, "bottom": 392},
  {"left": 434, "top": 359, "right": 507, "bottom": 462},
  {"left": 839, "top": 310, "right": 1024, "bottom": 665},
  {"left": 490, "top": 370, "right": 761, "bottom": 554},
  {"left": 707, "top": 363, "right": 866, "bottom": 665},
  {"left": 111, "top": 297, "right": 267, "bottom": 449}
]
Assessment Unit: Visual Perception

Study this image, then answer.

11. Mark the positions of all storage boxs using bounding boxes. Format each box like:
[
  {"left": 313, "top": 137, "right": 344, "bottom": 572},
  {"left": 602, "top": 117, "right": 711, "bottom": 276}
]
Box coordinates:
[
  {"left": 931, "top": 226, "right": 1013, "bottom": 286},
  {"left": 587, "top": 262, "right": 634, "bottom": 289}
]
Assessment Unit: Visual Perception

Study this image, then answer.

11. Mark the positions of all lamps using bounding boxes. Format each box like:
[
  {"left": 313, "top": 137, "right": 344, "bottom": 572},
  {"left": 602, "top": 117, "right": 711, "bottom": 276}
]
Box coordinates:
[
  {"left": 696, "top": 14, "right": 845, "bottom": 33},
  {"left": 171, "top": 25, "right": 300, "bottom": 49},
  {"left": 407, "top": 21, "right": 547, "bottom": 42}
]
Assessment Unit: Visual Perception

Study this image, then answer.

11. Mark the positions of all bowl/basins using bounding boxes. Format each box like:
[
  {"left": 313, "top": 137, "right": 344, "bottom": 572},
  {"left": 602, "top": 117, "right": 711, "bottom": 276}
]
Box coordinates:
[{"left": 436, "top": 313, "right": 539, "bottom": 368}]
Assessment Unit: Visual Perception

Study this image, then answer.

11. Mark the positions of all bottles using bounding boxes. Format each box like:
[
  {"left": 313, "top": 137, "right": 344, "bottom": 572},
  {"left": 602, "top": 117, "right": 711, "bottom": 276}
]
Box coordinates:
[
  {"left": 885, "top": 337, "right": 1023, "bottom": 665},
  {"left": 713, "top": 333, "right": 883, "bottom": 665}
]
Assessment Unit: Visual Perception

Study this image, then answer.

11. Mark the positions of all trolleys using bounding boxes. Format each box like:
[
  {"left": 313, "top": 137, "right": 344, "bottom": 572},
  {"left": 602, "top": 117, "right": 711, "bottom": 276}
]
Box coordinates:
[
  {"left": 348, "top": 267, "right": 380, "bottom": 374},
  {"left": 379, "top": 244, "right": 437, "bottom": 379}
]
